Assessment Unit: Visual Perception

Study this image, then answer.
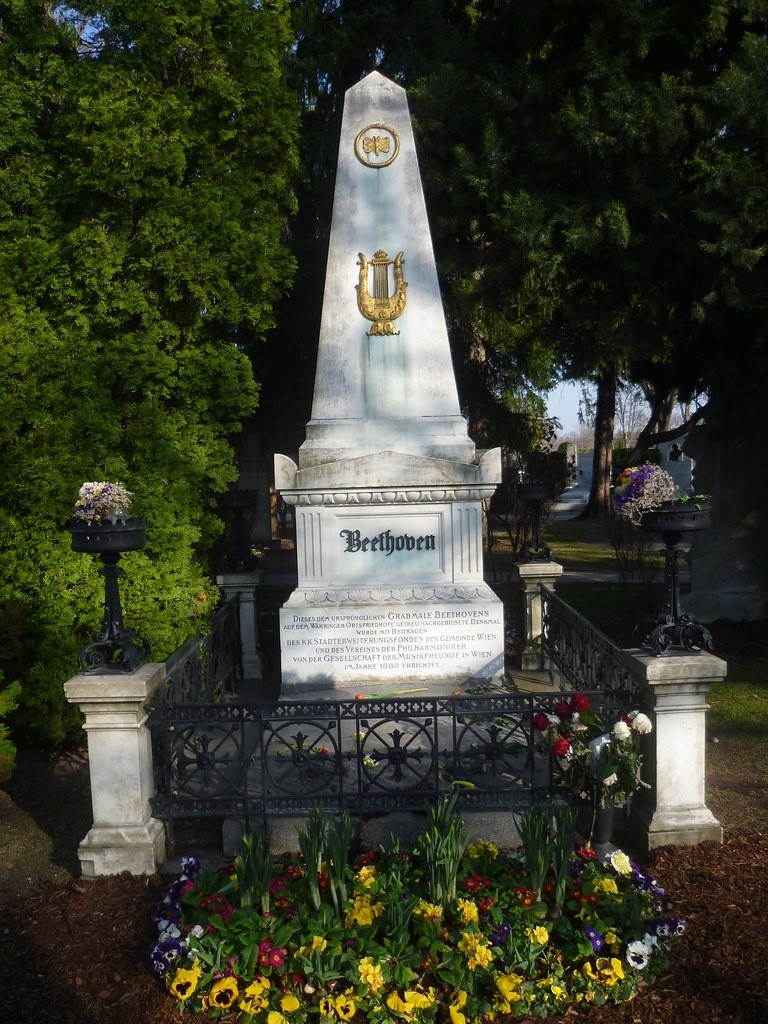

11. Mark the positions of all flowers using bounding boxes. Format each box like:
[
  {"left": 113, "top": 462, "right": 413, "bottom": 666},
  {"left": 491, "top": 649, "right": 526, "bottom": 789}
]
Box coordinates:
[
  {"left": 75, "top": 482, "right": 135, "bottom": 526},
  {"left": 148, "top": 690, "right": 690, "bottom": 1024},
  {"left": 612, "top": 462, "right": 673, "bottom": 527}
]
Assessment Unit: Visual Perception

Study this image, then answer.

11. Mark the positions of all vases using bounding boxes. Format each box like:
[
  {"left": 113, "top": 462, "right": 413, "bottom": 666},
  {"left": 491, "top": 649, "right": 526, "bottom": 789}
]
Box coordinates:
[
  {"left": 517, "top": 484, "right": 555, "bottom": 564},
  {"left": 68, "top": 514, "right": 153, "bottom": 676},
  {"left": 632, "top": 493, "right": 714, "bottom": 658},
  {"left": 212, "top": 488, "right": 258, "bottom": 573},
  {"left": 574, "top": 783, "right": 615, "bottom": 849}
]
